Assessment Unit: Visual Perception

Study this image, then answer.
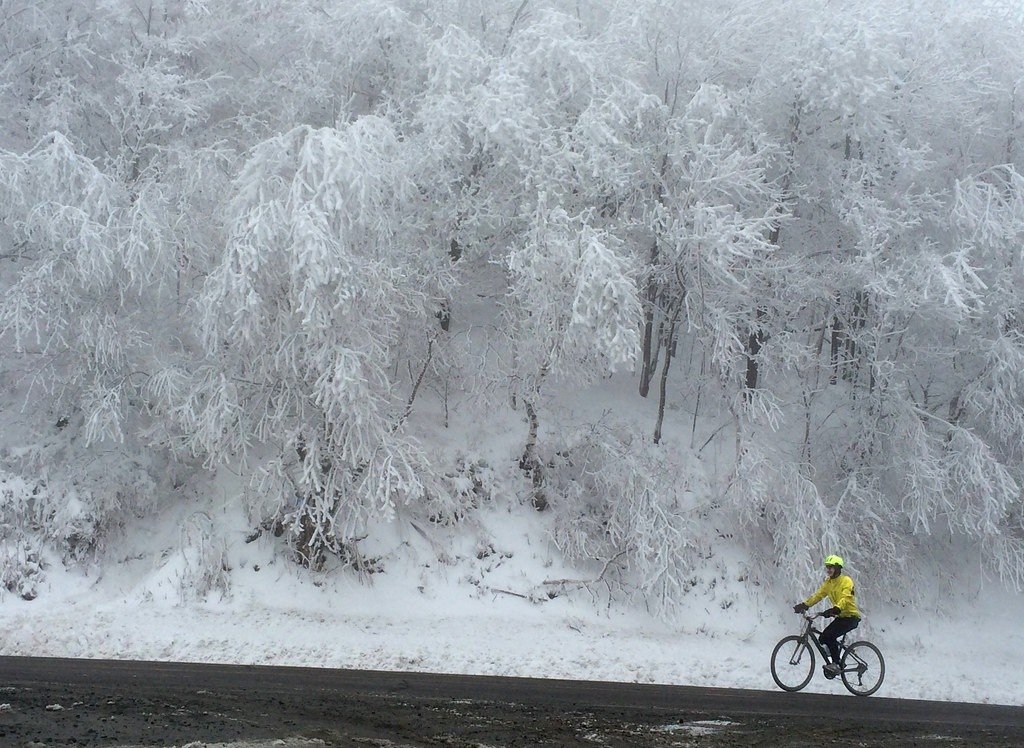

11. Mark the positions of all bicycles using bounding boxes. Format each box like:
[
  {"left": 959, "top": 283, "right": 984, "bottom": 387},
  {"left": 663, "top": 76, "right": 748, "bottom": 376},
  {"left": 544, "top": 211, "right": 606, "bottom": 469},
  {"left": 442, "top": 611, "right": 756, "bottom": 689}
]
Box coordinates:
[{"left": 771, "top": 607, "right": 886, "bottom": 697}]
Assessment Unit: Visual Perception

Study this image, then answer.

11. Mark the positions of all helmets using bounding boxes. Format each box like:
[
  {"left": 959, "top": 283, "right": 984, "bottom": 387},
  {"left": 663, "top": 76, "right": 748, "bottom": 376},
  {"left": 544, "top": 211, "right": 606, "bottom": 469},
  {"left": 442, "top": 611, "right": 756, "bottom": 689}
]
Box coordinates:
[{"left": 824, "top": 555, "right": 843, "bottom": 569}]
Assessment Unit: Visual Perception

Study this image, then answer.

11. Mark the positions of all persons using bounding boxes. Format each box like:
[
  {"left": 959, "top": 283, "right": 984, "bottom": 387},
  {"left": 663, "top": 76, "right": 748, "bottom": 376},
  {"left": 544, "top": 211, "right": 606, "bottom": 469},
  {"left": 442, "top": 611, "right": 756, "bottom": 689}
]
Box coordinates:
[{"left": 792, "top": 555, "right": 862, "bottom": 659}]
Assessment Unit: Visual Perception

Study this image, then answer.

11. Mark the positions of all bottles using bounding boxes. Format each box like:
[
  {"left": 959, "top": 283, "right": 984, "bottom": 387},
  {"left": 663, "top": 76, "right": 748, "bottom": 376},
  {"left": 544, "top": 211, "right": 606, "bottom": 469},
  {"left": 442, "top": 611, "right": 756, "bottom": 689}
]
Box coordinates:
[{"left": 823, "top": 645, "right": 831, "bottom": 656}]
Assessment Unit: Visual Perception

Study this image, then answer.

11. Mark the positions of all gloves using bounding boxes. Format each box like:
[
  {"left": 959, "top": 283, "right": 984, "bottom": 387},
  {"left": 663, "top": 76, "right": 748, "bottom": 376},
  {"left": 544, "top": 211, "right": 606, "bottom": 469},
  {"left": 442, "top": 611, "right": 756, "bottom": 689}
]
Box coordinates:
[
  {"left": 823, "top": 606, "right": 841, "bottom": 618},
  {"left": 794, "top": 603, "right": 809, "bottom": 613}
]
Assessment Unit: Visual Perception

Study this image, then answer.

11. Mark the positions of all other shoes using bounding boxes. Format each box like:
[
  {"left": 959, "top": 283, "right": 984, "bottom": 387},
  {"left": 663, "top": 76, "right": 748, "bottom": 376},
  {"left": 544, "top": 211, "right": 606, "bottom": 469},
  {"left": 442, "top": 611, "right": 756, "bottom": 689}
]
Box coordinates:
[
  {"left": 825, "top": 645, "right": 831, "bottom": 657},
  {"left": 822, "top": 662, "right": 841, "bottom": 674}
]
